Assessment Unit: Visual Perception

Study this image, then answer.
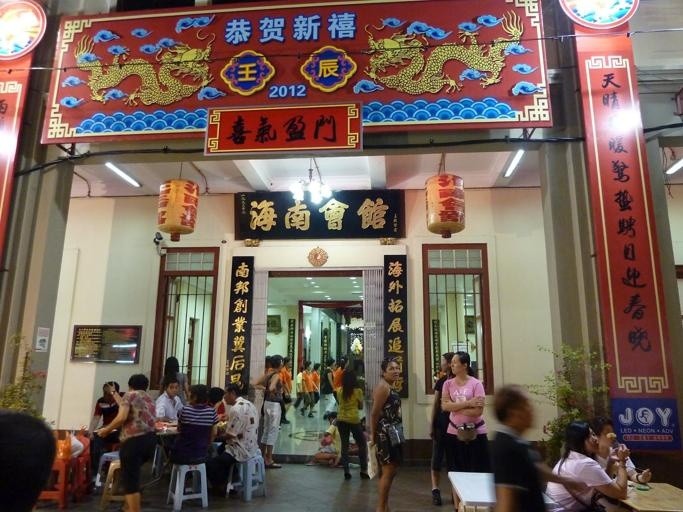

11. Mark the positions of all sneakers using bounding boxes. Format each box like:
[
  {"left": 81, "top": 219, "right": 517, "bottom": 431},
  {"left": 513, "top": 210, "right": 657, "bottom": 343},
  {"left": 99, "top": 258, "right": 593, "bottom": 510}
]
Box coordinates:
[
  {"left": 360, "top": 472, "right": 370, "bottom": 479},
  {"left": 344, "top": 472, "right": 352, "bottom": 480},
  {"left": 431, "top": 488, "right": 443, "bottom": 505}
]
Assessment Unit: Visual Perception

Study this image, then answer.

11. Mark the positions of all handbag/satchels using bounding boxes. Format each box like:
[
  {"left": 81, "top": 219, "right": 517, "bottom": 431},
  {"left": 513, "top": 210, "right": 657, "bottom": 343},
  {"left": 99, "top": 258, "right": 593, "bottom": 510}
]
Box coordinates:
[
  {"left": 386, "top": 423, "right": 407, "bottom": 447},
  {"left": 455, "top": 422, "right": 478, "bottom": 441}
]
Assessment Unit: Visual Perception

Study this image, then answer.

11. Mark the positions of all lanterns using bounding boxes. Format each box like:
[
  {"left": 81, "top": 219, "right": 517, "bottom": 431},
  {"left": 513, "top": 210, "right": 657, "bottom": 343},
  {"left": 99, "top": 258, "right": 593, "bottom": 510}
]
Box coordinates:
[
  {"left": 422, "top": 173, "right": 465, "bottom": 238},
  {"left": 157, "top": 179, "right": 199, "bottom": 242}
]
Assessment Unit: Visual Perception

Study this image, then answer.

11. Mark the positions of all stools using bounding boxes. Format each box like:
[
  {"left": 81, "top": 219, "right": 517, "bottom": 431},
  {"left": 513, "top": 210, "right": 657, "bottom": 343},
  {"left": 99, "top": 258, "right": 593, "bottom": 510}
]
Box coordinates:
[{"left": 36, "top": 416, "right": 270, "bottom": 512}]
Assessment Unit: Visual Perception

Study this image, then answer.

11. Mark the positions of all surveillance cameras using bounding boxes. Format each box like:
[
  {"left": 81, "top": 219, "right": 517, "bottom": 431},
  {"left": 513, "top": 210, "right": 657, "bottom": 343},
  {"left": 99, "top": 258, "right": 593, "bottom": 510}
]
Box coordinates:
[{"left": 156, "top": 232, "right": 163, "bottom": 240}]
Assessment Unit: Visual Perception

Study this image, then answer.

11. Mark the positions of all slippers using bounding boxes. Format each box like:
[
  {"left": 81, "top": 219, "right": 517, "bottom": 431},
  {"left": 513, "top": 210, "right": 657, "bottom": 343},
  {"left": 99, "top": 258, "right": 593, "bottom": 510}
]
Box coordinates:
[{"left": 263, "top": 460, "right": 283, "bottom": 468}]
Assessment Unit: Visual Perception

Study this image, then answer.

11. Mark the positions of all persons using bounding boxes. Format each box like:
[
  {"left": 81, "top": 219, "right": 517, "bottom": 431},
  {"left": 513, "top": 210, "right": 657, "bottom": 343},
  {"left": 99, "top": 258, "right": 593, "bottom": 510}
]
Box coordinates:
[
  {"left": 591, "top": 416, "right": 652, "bottom": 511},
  {"left": 425, "top": 352, "right": 454, "bottom": 505},
  {"left": 305, "top": 411, "right": 340, "bottom": 465},
  {"left": 254, "top": 354, "right": 287, "bottom": 468},
  {"left": 368, "top": 357, "right": 406, "bottom": 511},
  {"left": 546, "top": 419, "right": 630, "bottom": 511},
  {"left": 266, "top": 353, "right": 349, "bottom": 418},
  {"left": 89, "top": 355, "right": 263, "bottom": 512},
  {"left": 440, "top": 350, "right": 485, "bottom": 476},
  {"left": 488, "top": 383, "right": 548, "bottom": 512},
  {"left": 337, "top": 371, "right": 371, "bottom": 479},
  {"left": 0, "top": 411, "right": 56, "bottom": 510}
]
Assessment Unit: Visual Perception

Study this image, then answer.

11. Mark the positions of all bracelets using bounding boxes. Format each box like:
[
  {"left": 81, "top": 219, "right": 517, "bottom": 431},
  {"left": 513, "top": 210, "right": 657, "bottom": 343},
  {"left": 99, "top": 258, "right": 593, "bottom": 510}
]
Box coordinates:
[
  {"left": 616, "top": 461, "right": 626, "bottom": 467},
  {"left": 635, "top": 472, "right": 641, "bottom": 482}
]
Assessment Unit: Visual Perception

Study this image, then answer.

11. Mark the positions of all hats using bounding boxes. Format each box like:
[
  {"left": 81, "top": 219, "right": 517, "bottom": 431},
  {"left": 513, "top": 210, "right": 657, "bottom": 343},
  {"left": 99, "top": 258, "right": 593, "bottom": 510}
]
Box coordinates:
[{"left": 562, "top": 421, "right": 590, "bottom": 450}]
